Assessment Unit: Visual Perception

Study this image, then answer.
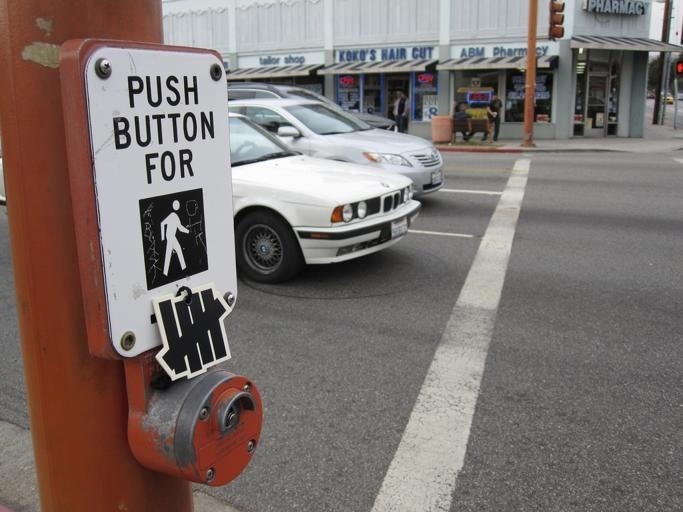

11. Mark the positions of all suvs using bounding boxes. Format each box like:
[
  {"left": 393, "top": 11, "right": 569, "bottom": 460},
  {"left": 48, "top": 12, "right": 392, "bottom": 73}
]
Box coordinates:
[{"left": 226, "top": 83, "right": 399, "bottom": 132}]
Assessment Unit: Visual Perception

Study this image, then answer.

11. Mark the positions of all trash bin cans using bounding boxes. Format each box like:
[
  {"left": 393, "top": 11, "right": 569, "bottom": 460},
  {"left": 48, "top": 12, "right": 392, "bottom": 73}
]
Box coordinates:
[{"left": 431, "top": 115, "right": 453, "bottom": 145}]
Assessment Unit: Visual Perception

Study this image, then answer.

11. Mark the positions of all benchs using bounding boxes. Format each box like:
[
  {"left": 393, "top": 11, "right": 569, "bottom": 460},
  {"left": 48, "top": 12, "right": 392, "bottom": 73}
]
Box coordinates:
[{"left": 450, "top": 116, "right": 494, "bottom": 144}]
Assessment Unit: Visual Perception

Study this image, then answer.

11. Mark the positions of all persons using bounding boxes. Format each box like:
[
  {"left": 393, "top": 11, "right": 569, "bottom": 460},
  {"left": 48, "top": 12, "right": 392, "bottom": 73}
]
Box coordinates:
[
  {"left": 479, "top": 92, "right": 502, "bottom": 140},
  {"left": 392, "top": 88, "right": 409, "bottom": 133},
  {"left": 454, "top": 103, "right": 476, "bottom": 141}
]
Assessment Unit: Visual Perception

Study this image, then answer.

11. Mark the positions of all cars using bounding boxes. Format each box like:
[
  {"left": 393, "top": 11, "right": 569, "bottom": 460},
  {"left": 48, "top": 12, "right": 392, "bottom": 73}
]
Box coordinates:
[
  {"left": 227, "top": 111, "right": 422, "bottom": 286},
  {"left": 226, "top": 98, "right": 446, "bottom": 199},
  {"left": 647, "top": 89, "right": 683, "bottom": 105}
]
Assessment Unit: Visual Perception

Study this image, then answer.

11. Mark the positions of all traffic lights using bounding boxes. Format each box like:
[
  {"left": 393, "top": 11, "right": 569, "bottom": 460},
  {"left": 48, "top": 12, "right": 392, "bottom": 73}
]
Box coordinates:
[
  {"left": 548, "top": 0, "right": 565, "bottom": 39},
  {"left": 675, "top": 61, "right": 682, "bottom": 76}
]
